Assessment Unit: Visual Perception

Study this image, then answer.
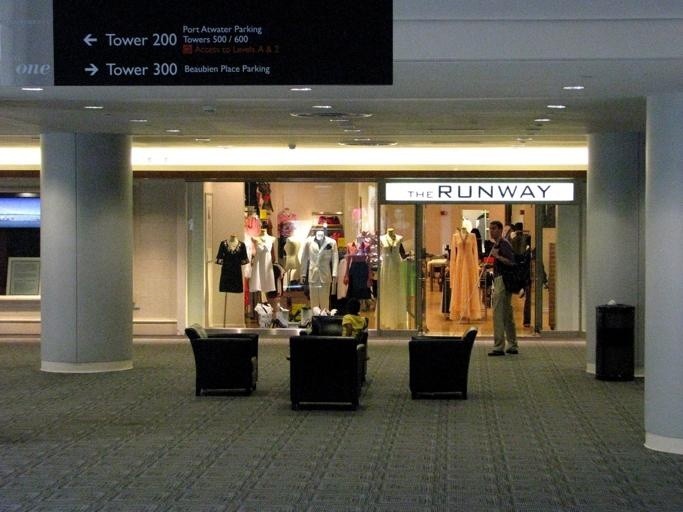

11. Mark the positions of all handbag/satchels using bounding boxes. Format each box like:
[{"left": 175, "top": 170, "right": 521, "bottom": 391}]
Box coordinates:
[
  {"left": 500, "top": 262, "right": 532, "bottom": 294},
  {"left": 311, "top": 314, "right": 343, "bottom": 337}
]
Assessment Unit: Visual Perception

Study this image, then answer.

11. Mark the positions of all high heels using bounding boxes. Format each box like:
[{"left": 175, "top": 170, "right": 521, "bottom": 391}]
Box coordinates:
[
  {"left": 271, "top": 318, "right": 287, "bottom": 328},
  {"left": 298, "top": 322, "right": 311, "bottom": 329}
]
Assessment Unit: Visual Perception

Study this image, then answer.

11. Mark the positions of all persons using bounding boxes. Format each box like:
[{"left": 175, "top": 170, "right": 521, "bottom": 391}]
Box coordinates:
[
  {"left": 248, "top": 227, "right": 279, "bottom": 296},
  {"left": 448, "top": 226, "right": 481, "bottom": 322},
  {"left": 299, "top": 230, "right": 339, "bottom": 312},
  {"left": 342, "top": 236, "right": 374, "bottom": 302},
  {"left": 479, "top": 220, "right": 521, "bottom": 356},
  {"left": 264, "top": 262, "right": 286, "bottom": 328},
  {"left": 214, "top": 232, "right": 249, "bottom": 295},
  {"left": 373, "top": 227, "right": 411, "bottom": 329},
  {"left": 469, "top": 222, "right": 549, "bottom": 329},
  {"left": 283, "top": 235, "right": 302, "bottom": 271}
]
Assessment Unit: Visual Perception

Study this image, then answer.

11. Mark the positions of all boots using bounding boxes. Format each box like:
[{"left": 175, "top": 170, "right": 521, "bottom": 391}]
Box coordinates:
[{"left": 289, "top": 309, "right": 301, "bottom": 322}]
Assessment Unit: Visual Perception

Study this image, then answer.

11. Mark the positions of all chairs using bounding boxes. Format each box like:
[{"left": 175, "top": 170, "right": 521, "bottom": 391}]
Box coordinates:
[
  {"left": 300, "top": 315, "right": 368, "bottom": 382},
  {"left": 409, "top": 327, "right": 477, "bottom": 399},
  {"left": 290, "top": 336, "right": 365, "bottom": 411},
  {"left": 185, "top": 323, "right": 258, "bottom": 396}
]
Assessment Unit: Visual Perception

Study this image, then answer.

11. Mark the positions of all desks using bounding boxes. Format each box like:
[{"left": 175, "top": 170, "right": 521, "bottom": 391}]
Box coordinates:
[{"left": 429, "top": 258, "right": 448, "bottom": 292}]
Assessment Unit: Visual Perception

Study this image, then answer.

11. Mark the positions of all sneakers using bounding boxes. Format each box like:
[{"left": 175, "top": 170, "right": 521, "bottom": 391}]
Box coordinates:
[
  {"left": 487, "top": 350, "right": 505, "bottom": 356},
  {"left": 506, "top": 348, "right": 518, "bottom": 354}
]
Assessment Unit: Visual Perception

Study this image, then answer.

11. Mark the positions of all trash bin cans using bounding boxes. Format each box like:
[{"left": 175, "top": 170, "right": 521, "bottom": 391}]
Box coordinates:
[{"left": 595, "top": 303, "right": 635, "bottom": 381}]
